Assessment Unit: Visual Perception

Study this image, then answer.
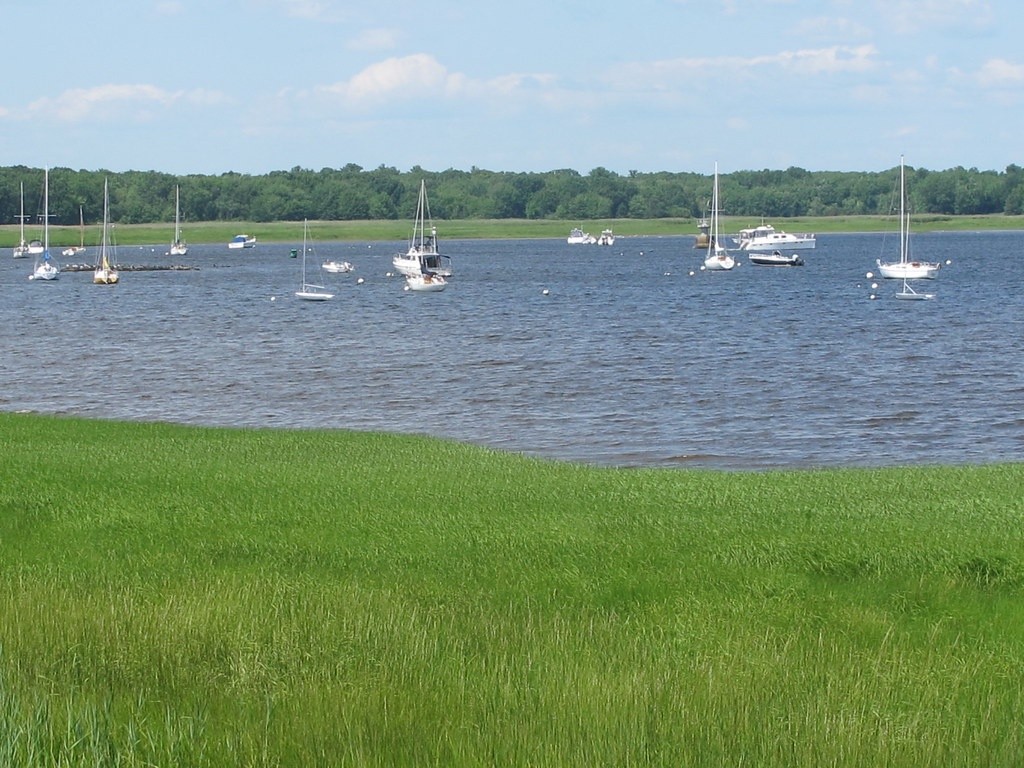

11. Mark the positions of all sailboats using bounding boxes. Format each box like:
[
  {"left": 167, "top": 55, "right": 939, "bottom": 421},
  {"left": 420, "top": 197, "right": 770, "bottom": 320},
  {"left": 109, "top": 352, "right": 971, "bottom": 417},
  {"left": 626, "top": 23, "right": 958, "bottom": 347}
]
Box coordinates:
[
  {"left": 13, "top": 182, "right": 31, "bottom": 258},
  {"left": 702, "top": 159, "right": 735, "bottom": 269},
  {"left": 170, "top": 184, "right": 189, "bottom": 255},
  {"left": 92, "top": 177, "right": 121, "bottom": 284},
  {"left": 894, "top": 208, "right": 936, "bottom": 300},
  {"left": 27, "top": 164, "right": 61, "bottom": 280},
  {"left": 62, "top": 205, "right": 87, "bottom": 256},
  {"left": 878, "top": 153, "right": 941, "bottom": 280},
  {"left": 392, "top": 179, "right": 453, "bottom": 277},
  {"left": 295, "top": 218, "right": 336, "bottom": 300}
]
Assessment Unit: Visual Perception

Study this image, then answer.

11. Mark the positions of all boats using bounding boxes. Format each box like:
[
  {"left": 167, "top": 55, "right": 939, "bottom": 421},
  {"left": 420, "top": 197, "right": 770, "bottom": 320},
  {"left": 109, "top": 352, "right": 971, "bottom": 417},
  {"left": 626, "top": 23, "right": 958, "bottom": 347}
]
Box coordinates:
[
  {"left": 404, "top": 273, "right": 448, "bottom": 292},
  {"left": 748, "top": 250, "right": 804, "bottom": 266},
  {"left": 739, "top": 230, "right": 816, "bottom": 251},
  {"left": 228, "top": 234, "right": 256, "bottom": 249},
  {"left": 731, "top": 224, "right": 776, "bottom": 244},
  {"left": 567, "top": 227, "right": 597, "bottom": 245},
  {"left": 321, "top": 258, "right": 354, "bottom": 273},
  {"left": 596, "top": 229, "right": 615, "bottom": 246},
  {"left": 27, "top": 239, "right": 44, "bottom": 254},
  {"left": 694, "top": 210, "right": 716, "bottom": 248}
]
